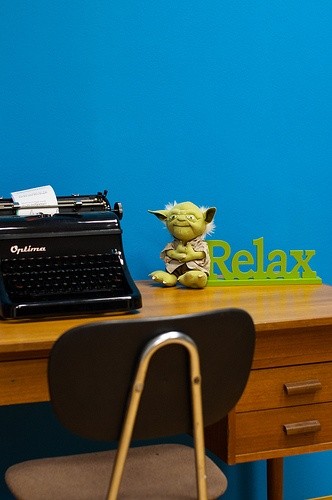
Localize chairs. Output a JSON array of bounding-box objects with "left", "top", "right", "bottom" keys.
[{"left": 4, "top": 306, "right": 257, "bottom": 499}]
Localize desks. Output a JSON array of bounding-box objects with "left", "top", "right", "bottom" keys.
[{"left": 0, "top": 272, "right": 331, "bottom": 500}]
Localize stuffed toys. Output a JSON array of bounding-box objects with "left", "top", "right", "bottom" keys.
[{"left": 147, "top": 200, "right": 218, "bottom": 289}]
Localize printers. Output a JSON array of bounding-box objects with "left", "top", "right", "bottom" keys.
[{"left": 1, "top": 185, "right": 143, "bottom": 317}]
